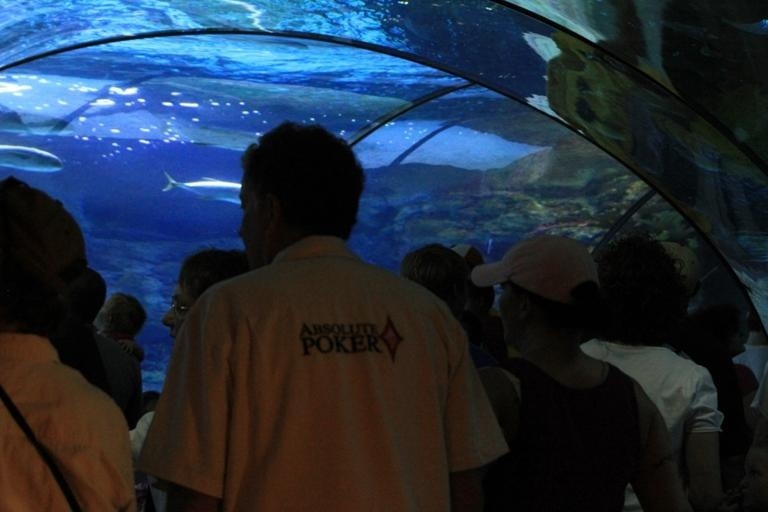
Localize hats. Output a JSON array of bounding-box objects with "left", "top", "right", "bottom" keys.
[
  {"left": 2, "top": 175, "right": 85, "bottom": 297},
  {"left": 472, "top": 233, "right": 601, "bottom": 305}
]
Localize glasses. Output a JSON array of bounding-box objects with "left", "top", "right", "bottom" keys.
[{"left": 170, "top": 298, "right": 189, "bottom": 319}]
